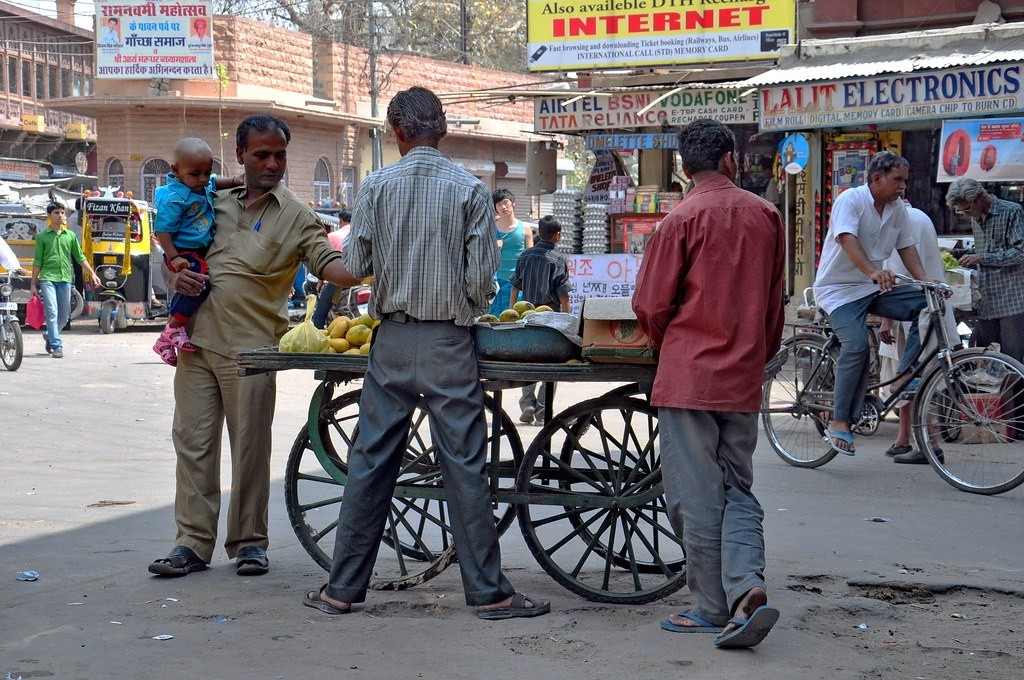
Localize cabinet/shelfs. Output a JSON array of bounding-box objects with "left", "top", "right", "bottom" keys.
[{"left": 734, "top": 127, "right": 780, "bottom": 193}]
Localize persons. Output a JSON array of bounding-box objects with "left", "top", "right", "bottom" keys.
[
  {"left": 300, "top": 86, "right": 555, "bottom": 620},
  {"left": 66, "top": 197, "right": 84, "bottom": 297},
  {"left": 311, "top": 212, "right": 352, "bottom": 329},
  {"left": 190, "top": 19, "right": 211, "bottom": 44},
  {"left": 946, "top": 177, "right": 1024, "bottom": 442},
  {"left": 30, "top": 202, "right": 101, "bottom": 357},
  {"left": 150, "top": 137, "right": 245, "bottom": 368},
  {"left": 0, "top": 235, "right": 29, "bottom": 277},
  {"left": 487, "top": 188, "right": 533, "bottom": 321},
  {"left": 814, "top": 153, "right": 954, "bottom": 456},
  {"left": 101, "top": 18, "right": 119, "bottom": 44},
  {"left": 881, "top": 195, "right": 960, "bottom": 463},
  {"left": 632, "top": 119, "right": 784, "bottom": 649},
  {"left": 146, "top": 114, "right": 363, "bottom": 577},
  {"left": 757, "top": 157, "right": 786, "bottom": 214},
  {"left": 506, "top": 214, "right": 572, "bottom": 427}
]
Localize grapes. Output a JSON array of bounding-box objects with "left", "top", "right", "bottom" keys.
[{"left": 939, "top": 250, "right": 958, "bottom": 270}]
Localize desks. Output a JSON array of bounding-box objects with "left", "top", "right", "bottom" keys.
[{"left": 610, "top": 211, "right": 668, "bottom": 254}]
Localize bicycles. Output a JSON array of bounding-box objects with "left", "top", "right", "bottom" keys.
[{"left": 759, "top": 272, "right": 1024, "bottom": 496}]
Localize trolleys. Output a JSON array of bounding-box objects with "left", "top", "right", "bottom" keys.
[{"left": 240, "top": 343, "right": 691, "bottom": 609}]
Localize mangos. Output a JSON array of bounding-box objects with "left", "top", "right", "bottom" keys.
[
  {"left": 319, "top": 314, "right": 382, "bottom": 355},
  {"left": 480, "top": 301, "right": 553, "bottom": 323},
  {"left": 567, "top": 359, "right": 582, "bottom": 365}
]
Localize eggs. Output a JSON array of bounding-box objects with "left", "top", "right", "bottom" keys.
[{"left": 554, "top": 189, "right": 610, "bottom": 254}]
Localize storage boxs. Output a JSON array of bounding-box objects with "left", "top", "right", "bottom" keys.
[
  {"left": 957, "top": 371, "right": 1019, "bottom": 444},
  {"left": 582, "top": 297, "right": 657, "bottom": 365}
]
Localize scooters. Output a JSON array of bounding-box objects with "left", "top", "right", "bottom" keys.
[{"left": 0, "top": 270, "right": 28, "bottom": 371}]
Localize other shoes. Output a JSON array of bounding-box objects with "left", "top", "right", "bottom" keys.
[
  {"left": 520, "top": 406, "right": 535, "bottom": 422},
  {"left": 535, "top": 421, "right": 544, "bottom": 426},
  {"left": 894, "top": 448, "right": 944, "bottom": 464},
  {"left": 53, "top": 347, "right": 63, "bottom": 358},
  {"left": 43, "top": 333, "right": 53, "bottom": 354},
  {"left": 886, "top": 443, "right": 913, "bottom": 457}
]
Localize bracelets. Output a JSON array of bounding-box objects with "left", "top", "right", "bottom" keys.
[
  {"left": 170, "top": 254, "right": 181, "bottom": 261},
  {"left": 232, "top": 176, "right": 236, "bottom": 185}
]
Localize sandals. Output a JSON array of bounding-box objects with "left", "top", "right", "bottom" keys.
[
  {"left": 153, "top": 338, "right": 177, "bottom": 366},
  {"left": 161, "top": 324, "right": 197, "bottom": 352}
]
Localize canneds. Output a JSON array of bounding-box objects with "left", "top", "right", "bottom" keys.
[{"left": 839, "top": 164, "right": 857, "bottom": 183}]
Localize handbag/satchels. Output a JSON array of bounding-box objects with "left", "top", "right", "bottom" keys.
[
  {"left": 27, "top": 295, "right": 44, "bottom": 330},
  {"left": 279, "top": 294, "right": 329, "bottom": 353}
]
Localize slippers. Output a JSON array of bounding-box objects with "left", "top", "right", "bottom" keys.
[
  {"left": 824, "top": 429, "right": 855, "bottom": 456},
  {"left": 715, "top": 606, "right": 780, "bottom": 647},
  {"left": 148, "top": 546, "right": 207, "bottom": 576},
  {"left": 893, "top": 379, "right": 941, "bottom": 398},
  {"left": 478, "top": 591, "right": 550, "bottom": 618},
  {"left": 236, "top": 546, "right": 269, "bottom": 574},
  {"left": 661, "top": 609, "right": 725, "bottom": 632},
  {"left": 303, "top": 584, "right": 343, "bottom": 614}
]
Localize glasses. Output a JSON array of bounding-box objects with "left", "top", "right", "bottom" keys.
[{"left": 956, "top": 201, "right": 973, "bottom": 214}]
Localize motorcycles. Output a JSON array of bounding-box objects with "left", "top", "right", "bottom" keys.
[{"left": 74, "top": 190, "right": 168, "bottom": 334}]
